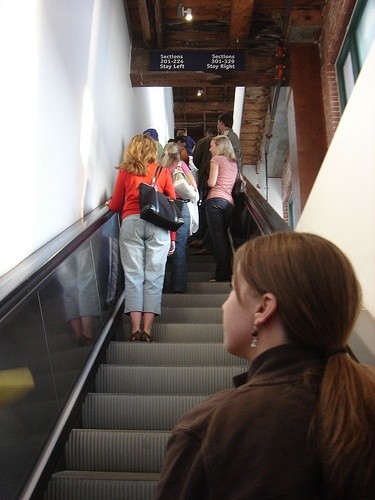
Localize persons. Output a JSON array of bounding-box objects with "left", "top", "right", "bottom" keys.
[
  {"left": 190, "top": 127, "right": 218, "bottom": 254},
  {"left": 106, "top": 133, "right": 175, "bottom": 342},
  {"left": 217, "top": 114, "right": 240, "bottom": 160},
  {"left": 205, "top": 138, "right": 238, "bottom": 282},
  {"left": 155, "top": 232, "right": 375, "bottom": 500},
  {"left": 58, "top": 229, "right": 103, "bottom": 347},
  {"left": 163, "top": 143, "right": 199, "bottom": 293},
  {"left": 168, "top": 129, "right": 198, "bottom": 177},
  {"left": 143, "top": 129, "right": 164, "bottom": 162}
]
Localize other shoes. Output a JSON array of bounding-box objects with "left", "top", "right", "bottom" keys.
[
  {"left": 163, "top": 290, "right": 184, "bottom": 294},
  {"left": 208, "top": 278, "right": 218, "bottom": 283},
  {"left": 128, "top": 330, "right": 153, "bottom": 343},
  {"left": 76, "top": 335, "right": 96, "bottom": 348},
  {"left": 191, "top": 248, "right": 212, "bottom": 255},
  {"left": 189, "top": 240, "right": 205, "bottom": 247}
]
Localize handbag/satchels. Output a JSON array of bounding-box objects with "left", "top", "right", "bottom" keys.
[
  {"left": 139, "top": 166, "right": 185, "bottom": 232},
  {"left": 172, "top": 160, "right": 200, "bottom": 204},
  {"left": 231, "top": 166, "right": 244, "bottom": 196}
]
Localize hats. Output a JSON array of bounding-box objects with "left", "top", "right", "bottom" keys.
[{"left": 143, "top": 129, "right": 158, "bottom": 141}]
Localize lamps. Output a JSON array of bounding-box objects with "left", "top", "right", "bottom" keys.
[
  {"left": 182, "top": 7, "right": 192, "bottom": 21},
  {"left": 197, "top": 90, "right": 203, "bottom": 96}
]
